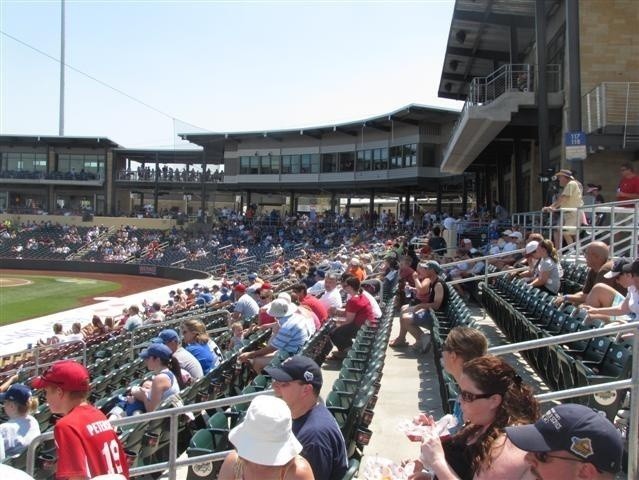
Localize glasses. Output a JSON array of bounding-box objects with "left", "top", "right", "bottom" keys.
[
  {"left": 458, "top": 390, "right": 496, "bottom": 402},
  {"left": 441, "top": 344, "right": 458, "bottom": 354}
]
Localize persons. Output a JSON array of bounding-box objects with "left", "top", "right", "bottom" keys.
[
  {"left": 516, "top": 68, "right": 534, "bottom": 91},
  {"left": 155, "top": 329, "right": 204, "bottom": 388},
  {"left": 345, "top": 259, "right": 365, "bottom": 282},
  {"left": 396, "top": 255, "right": 416, "bottom": 289},
  {"left": 44, "top": 361, "right": 129, "bottom": 476},
  {"left": 94, "top": 202, "right": 448, "bottom": 228},
  {"left": 176, "top": 318, "right": 214, "bottom": 376},
  {"left": 582, "top": 258, "right": 634, "bottom": 322},
  {"left": 273, "top": 354, "right": 350, "bottom": 476},
  {"left": 0, "top": 383, "right": 42, "bottom": 470},
  {"left": 316, "top": 226, "right": 431, "bottom": 264},
  {"left": 107, "top": 343, "right": 180, "bottom": 422},
  {"left": 553, "top": 241, "right": 611, "bottom": 310},
  {"left": 0, "top": 374, "right": 19, "bottom": 393},
  {"left": 123, "top": 306, "right": 143, "bottom": 332},
  {"left": 259, "top": 277, "right": 296, "bottom": 299},
  {"left": 542, "top": 170, "right": 584, "bottom": 256},
  {"left": 616, "top": 163, "right": 639, "bottom": 259},
  {"left": 444, "top": 214, "right": 462, "bottom": 229},
  {"left": 118, "top": 162, "right": 223, "bottom": 184},
  {"left": 420, "top": 356, "right": 535, "bottom": 476},
  {"left": 429, "top": 227, "right": 447, "bottom": 260},
  {"left": 141, "top": 299, "right": 166, "bottom": 324},
  {"left": 177, "top": 225, "right": 320, "bottom": 260},
  {"left": 527, "top": 239, "right": 560, "bottom": 296},
  {"left": 102, "top": 223, "right": 182, "bottom": 266},
  {"left": 238, "top": 298, "right": 307, "bottom": 378},
  {"left": 157, "top": 278, "right": 250, "bottom": 316},
  {"left": 62, "top": 323, "right": 84, "bottom": 341},
  {"left": 246, "top": 274, "right": 262, "bottom": 296},
  {"left": 306, "top": 269, "right": 326, "bottom": 296},
  {"left": 365, "top": 256, "right": 396, "bottom": 289},
  {"left": 448, "top": 228, "right": 542, "bottom": 275},
  {"left": 214, "top": 258, "right": 315, "bottom": 280},
  {"left": 413, "top": 325, "right": 488, "bottom": 438},
  {"left": 218, "top": 395, "right": 313, "bottom": 476},
  {"left": 328, "top": 276, "right": 375, "bottom": 357},
  {"left": 459, "top": 198, "right": 500, "bottom": 224},
  {"left": 324, "top": 158, "right": 356, "bottom": 173},
  {"left": 487, "top": 215, "right": 501, "bottom": 229},
  {"left": 1, "top": 166, "right": 99, "bottom": 182},
  {"left": 491, "top": 200, "right": 509, "bottom": 222},
  {"left": 525, "top": 240, "right": 541, "bottom": 259},
  {"left": 37, "top": 323, "right": 65, "bottom": 347},
  {"left": 227, "top": 321, "right": 255, "bottom": 352},
  {"left": 116, "top": 308, "right": 129, "bottom": 327},
  {"left": 390, "top": 261, "right": 449, "bottom": 354},
  {"left": 318, "top": 275, "right": 342, "bottom": 310},
  {"left": 576, "top": 257, "right": 638, "bottom": 343},
  {"left": 84, "top": 316, "right": 109, "bottom": 340},
  {"left": 291, "top": 284, "right": 330, "bottom": 325},
  {"left": 0, "top": 218, "right": 109, "bottom": 261},
  {"left": 1, "top": 193, "right": 94, "bottom": 217},
  {"left": 580, "top": 184, "right": 606, "bottom": 242},
  {"left": 451, "top": 251, "right": 485, "bottom": 306},
  {"left": 524, "top": 403, "right": 625, "bottom": 475},
  {"left": 197, "top": 322, "right": 223, "bottom": 367},
  {"left": 400, "top": 261, "right": 432, "bottom": 314},
  {"left": 226, "top": 284, "right": 260, "bottom": 330}
]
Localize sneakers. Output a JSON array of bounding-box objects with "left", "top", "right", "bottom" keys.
[{"left": 418, "top": 334, "right": 431, "bottom": 355}]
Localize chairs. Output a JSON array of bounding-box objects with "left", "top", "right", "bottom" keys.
[
  {"left": 472, "top": 262, "right": 635, "bottom": 428},
  {"left": 430, "top": 283, "right": 492, "bottom": 419},
  {"left": 0, "top": 307, "right": 335, "bottom": 480},
  {"left": 326, "top": 293, "right": 400, "bottom": 478}
]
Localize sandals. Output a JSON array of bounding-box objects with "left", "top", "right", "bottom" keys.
[{"left": 389, "top": 339, "right": 409, "bottom": 348}]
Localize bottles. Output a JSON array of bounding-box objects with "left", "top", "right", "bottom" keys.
[
  {"left": 405, "top": 281, "right": 412, "bottom": 297},
  {"left": 237, "top": 357, "right": 244, "bottom": 372}
]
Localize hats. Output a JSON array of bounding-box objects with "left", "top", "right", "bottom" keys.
[
  {"left": 153, "top": 329, "right": 179, "bottom": 343},
  {"left": 554, "top": 168, "right": 574, "bottom": 180},
  {"left": 461, "top": 238, "right": 472, "bottom": 245},
  {"left": 139, "top": 341, "right": 172, "bottom": 363},
  {"left": 418, "top": 259, "right": 442, "bottom": 276},
  {"left": 247, "top": 273, "right": 257, "bottom": 280},
  {"left": 603, "top": 258, "right": 631, "bottom": 279},
  {"left": 509, "top": 232, "right": 523, "bottom": 240},
  {"left": 233, "top": 284, "right": 247, "bottom": 292},
  {"left": 277, "top": 292, "right": 291, "bottom": 304},
  {"left": 488, "top": 231, "right": 499, "bottom": 240},
  {"left": 444, "top": 217, "right": 457, "bottom": 229},
  {"left": 623, "top": 258, "right": 639, "bottom": 275},
  {"left": 227, "top": 393, "right": 304, "bottom": 467},
  {"left": 265, "top": 298, "right": 293, "bottom": 318},
  {"left": 31, "top": 358, "right": 90, "bottom": 393},
  {"left": 262, "top": 354, "right": 323, "bottom": 386},
  {"left": 526, "top": 241, "right": 539, "bottom": 254},
  {"left": 502, "top": 401, "right": 623, "bottom": 475},
  {"left": 0, "top": 382, "right": 33, "bottom": 406},
  {"left": 503, "top": 229, "right": 513, "bottom": 235}
]
